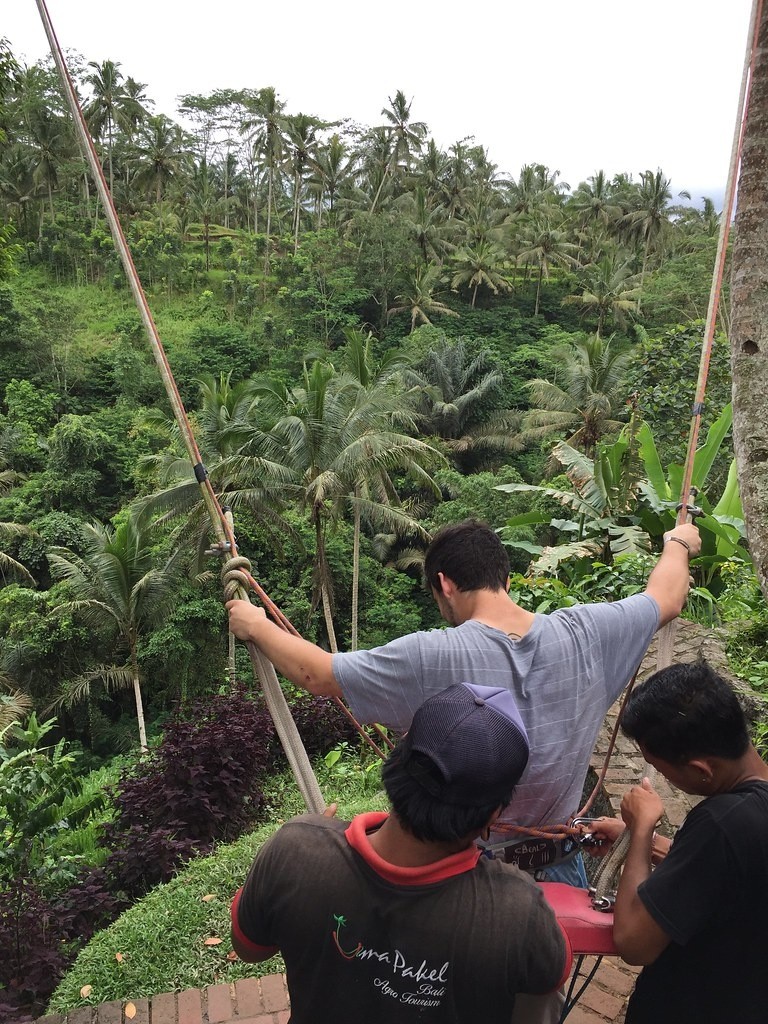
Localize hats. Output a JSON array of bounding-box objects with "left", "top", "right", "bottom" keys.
[{"left": 403, "top": 683, "right": 530, "bottom": 797}]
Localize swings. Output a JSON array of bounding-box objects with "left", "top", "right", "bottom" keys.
[{"left": 27, "top": 0, "right": 755, "bottom": 1024}]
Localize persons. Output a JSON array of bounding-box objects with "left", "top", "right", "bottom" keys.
[
  {"left": 223, "top": 519, "right": 703, "bottom": 889},
  {"left": 580, "top": 662, "right": 768, "bottom": 1024},
  {"left": 228, "top": 683, "right": 574, "bottom": 1024}
]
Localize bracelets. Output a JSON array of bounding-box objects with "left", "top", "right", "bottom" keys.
[
  {"left": 670, "top": 537, "right": 690, "bottom": 553},
  {"left": 650, "top": 831, "right": 658, "bottom": 858}
]
